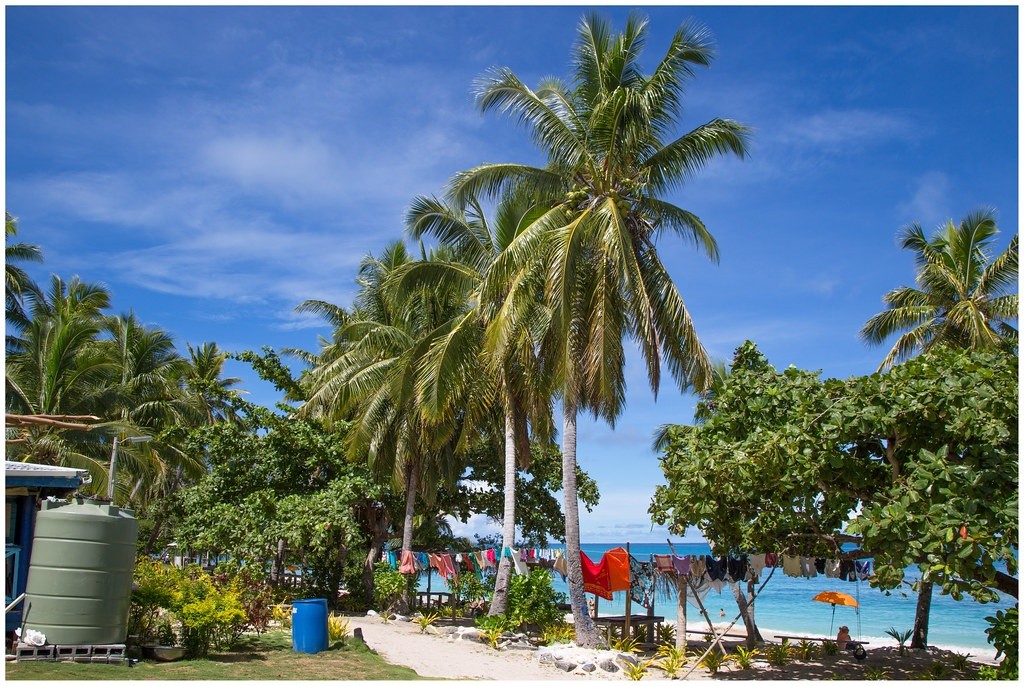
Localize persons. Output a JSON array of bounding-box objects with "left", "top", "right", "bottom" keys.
[
  {"left": 589, "top": 597, "right": 595, "bottom": 616},
  {"left": 720, "top": 608, "right": 725, "bottom": 616},
  {"left": 837, "top": 625, "right": 851, "bottom": 648}
]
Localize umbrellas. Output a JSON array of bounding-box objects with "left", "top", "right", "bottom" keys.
[{"left": 812, "top": 591, "right": 860, "bottom": 636}]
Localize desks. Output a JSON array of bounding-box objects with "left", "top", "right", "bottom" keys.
[
  {"left": 417, "top": 592, "right": 451, "bottom": 609},
  {"left": 592, "top": 615, "right": 665, "bottom": 643}
]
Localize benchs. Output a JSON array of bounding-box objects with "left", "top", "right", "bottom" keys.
[{"left": 774, "top": 635, "right": 869, "bottom": 654}]
